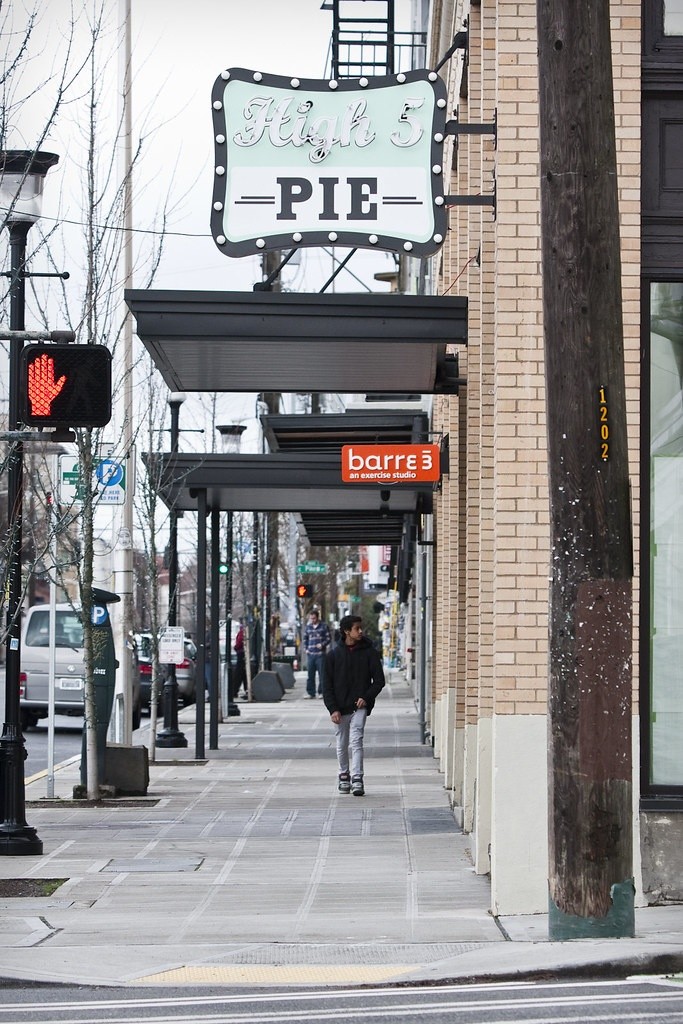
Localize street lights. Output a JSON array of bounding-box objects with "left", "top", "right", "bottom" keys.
[
  {"left": 1, "top": 137, "right": 56, "bottom": 860},
  {"left": 56, "top": 449, "right": 125, "bottom": 506}
]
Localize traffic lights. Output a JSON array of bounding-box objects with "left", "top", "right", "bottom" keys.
[
  {"left": 297, "top": 583, "right": 312, "bottom": 598},
  {"left": 16, "top": 336, "right": 115, "bottom": 429}
]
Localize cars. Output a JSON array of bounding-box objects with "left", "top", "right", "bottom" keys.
[{"left": 132, "top": 628, "right": 203, "bottom": 716}]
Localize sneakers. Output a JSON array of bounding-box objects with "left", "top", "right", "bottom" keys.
[
  {"left": 351, "top": 775, "right": 365, "bottom": 796},
  {"left": 338, "top": 772, "right": 351, "bottom": 794}
]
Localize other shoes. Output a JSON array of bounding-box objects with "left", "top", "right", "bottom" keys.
[{"left": 303, "top": 694, "right": 323, "bottom": 699}]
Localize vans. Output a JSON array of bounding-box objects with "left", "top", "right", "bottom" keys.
[{"left": 13, "top": 595, "right": 143, "bottom": 730}]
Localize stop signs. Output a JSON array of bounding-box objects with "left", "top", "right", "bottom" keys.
[{"left": 90, "top": 603, "right": 109, "bottom": 626}]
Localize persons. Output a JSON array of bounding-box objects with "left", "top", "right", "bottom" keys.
[
  {"left": 201, "top": 626, "right": 216, "bottom": 702},
  {"left": 330, "top": 623, "right": 339, "bottom": 644},
  {"left": 233, "top": 624, "right": 251, "bottom": 700},
  {"left": 373, "top": 633, "right": 383, "bottom": 662},
  {"left": 274, "top": 620, "right": 281, "bottom": 650},
  {"left": 305, "top": 611, "right": 330, "bottom": 702},
  {"left": 285, "top": 628, "right": 294, "bottom": 647},
  {"left": 321, "top": 615, "right": 385, "bottom": 795}
]
